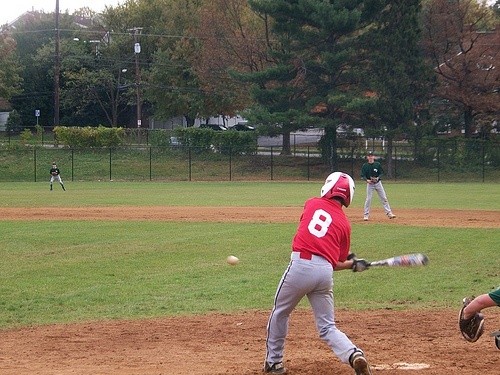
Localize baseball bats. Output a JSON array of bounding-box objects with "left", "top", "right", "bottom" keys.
[{"left": 365, "top": 253, "right": 429, "bottom": 267}]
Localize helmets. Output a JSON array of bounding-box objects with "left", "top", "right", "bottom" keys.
[{"left": 319, "top": 170, "right": 356, "bottom": 209}]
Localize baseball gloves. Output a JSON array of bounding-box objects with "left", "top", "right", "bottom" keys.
[
  {"left": 52, "top": 172, "right": 58, "bottom": 177},
  {"left": 369, "top": 176, "right": 380, "bottom": 184},
  {"left": 457, "top": 296, "right": 486, "bottom": 343}
]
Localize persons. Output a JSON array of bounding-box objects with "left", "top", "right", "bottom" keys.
[
  {"left": 49, "top": 162, "right": 66, "bottom": 191},
  {"left": 263, "top": 172, "right": 372, "bottom": 375},
  {"left": 458, "top": 286, "right": 500, "bottom": 349},
  {"left": 360, "top": 151, "right": 393, "bottom": 217}
]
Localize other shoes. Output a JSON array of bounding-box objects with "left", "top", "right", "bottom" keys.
[
  {"left": 363, "top": 215, "right": 369, "bottom": 221},
  {"left": 388, "top": 212, "right": 396, "bottom": 219}
]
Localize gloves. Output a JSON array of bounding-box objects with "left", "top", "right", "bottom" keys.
[
  {"left": 346, "top": 253, "right": 357, "bottom": 261},
  {"left": 351, "top": 258, "right": 370, "bottom": 273}
]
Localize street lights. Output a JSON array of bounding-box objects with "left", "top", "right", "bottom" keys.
[{"left": 126, "top": 26, "right": 144, "bottom": 144}]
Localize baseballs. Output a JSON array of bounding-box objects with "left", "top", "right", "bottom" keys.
[{"left": 226, "top": 255, "right": 239, "bottom": 266}]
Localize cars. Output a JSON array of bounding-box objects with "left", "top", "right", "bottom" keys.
[
  {"left": 198, "top": 124, "right": 229, "bottom": 131},
  {"left": 229, "top": 122, "right": 255, "bottom": 131},
  {"left": 167, "top": 136, "right": 182, "bottom": 147}
]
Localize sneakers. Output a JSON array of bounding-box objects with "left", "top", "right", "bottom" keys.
[
  {"left": 349, "top": 348, "right": 372, "bottom": 375},
  {"left": 263, "top": 358, "right": 286, "bottom": 374}
]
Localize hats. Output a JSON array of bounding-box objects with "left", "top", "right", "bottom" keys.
[{"left": 367, "top": 150, "right": 375, "bottom": 156}]
